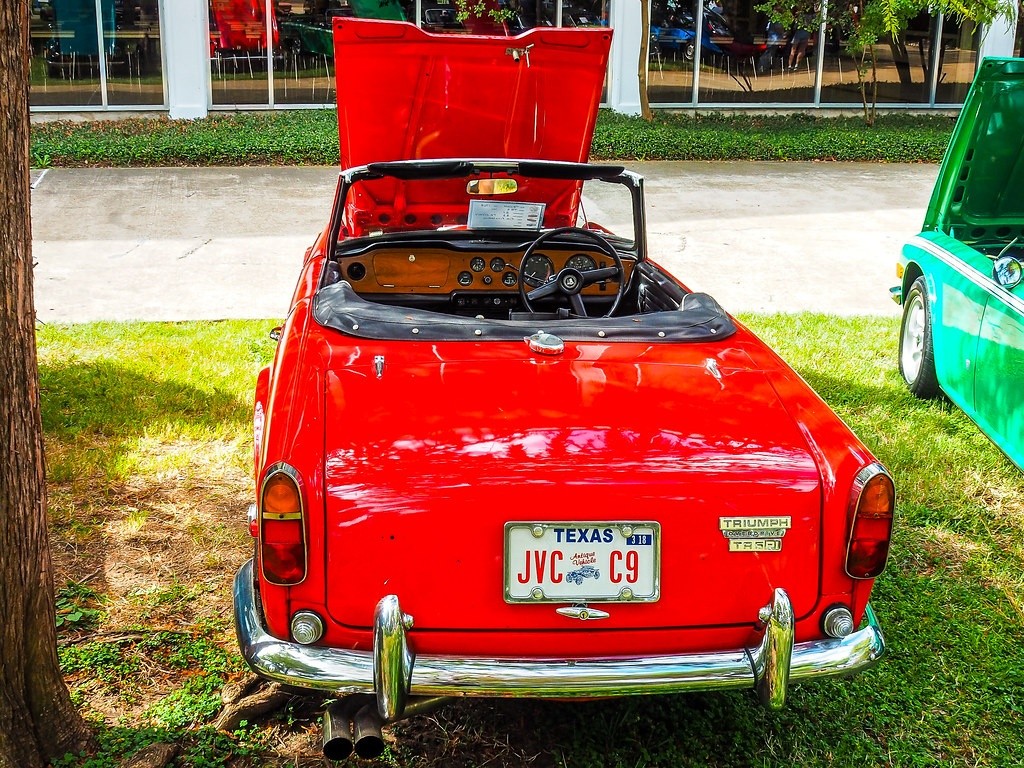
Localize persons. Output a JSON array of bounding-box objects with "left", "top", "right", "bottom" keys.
[{"left": 726, "top": 6, "right": 817, "bottom": 72}]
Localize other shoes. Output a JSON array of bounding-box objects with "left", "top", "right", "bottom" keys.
[
  {"left": 788, "top": 68, "right": 793, "bottom": 72},
  {"left": 794, "top": 67, "right": 798, "bottom": 72}
]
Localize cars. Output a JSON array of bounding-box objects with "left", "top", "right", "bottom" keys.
[
  {"left": 233, "top": 16, "right": 895, "bottom": 758},
  {"left": 899, "top": 54, "right": 1024, "bottom": 485},
  {"left": 650, "top": 4, "right": 731, "bottom": 61}
]
